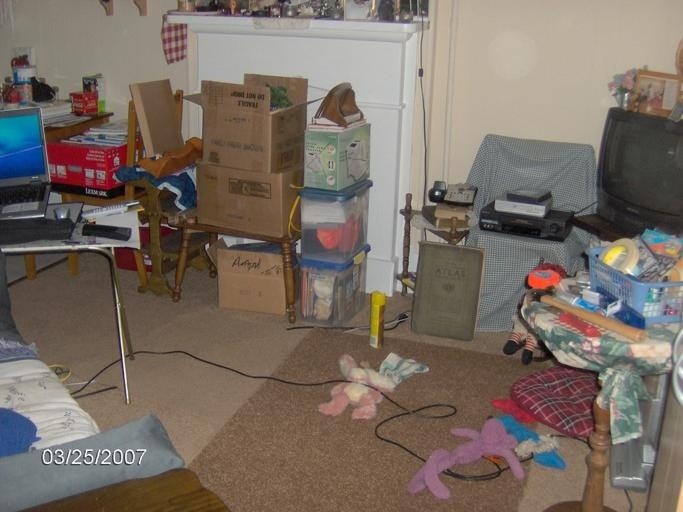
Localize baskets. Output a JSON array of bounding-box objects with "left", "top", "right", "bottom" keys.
[{"left": 584, "top": 247, "right": 683, "bottom": 326}]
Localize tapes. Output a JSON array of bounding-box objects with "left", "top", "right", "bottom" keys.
[{"left": 596, "top": 237, "right": 640, "bottom": 281}]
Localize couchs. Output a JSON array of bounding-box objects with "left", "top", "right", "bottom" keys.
[{"left": 0, "top": 250, "right": 183, "bottom": 511}]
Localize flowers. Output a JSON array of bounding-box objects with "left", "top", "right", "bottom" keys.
[{"left": 606, "top": 66, "right": 638, "bottom": 96}]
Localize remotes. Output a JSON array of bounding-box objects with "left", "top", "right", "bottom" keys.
[
  {"left": 81, "top": 202, "right": 127, "bottom": 219},
  {"left": 82, "top": 224, "right": 132, "bottom": 241}
]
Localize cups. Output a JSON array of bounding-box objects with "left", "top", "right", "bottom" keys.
[{"left": 574, "top": 270, "right": 591, "bottom": 291}]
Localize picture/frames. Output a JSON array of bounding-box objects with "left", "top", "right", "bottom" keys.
[{"left": 630, "top": 69, "right": 683, "bottom": 119}]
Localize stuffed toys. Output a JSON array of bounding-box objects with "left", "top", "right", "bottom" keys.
[
  {"left": 316, "top": 351, "right": 388, "bottom": 421},
  {"left": 504, "top": 311, "right": 539, "bottom": 364},
  {"left": 407, "top": 410, "right": 523, "bottom": 498}
]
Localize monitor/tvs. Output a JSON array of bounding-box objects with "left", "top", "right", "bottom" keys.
[{"left": 596, "top": 106, "right": 683, "bottom": 235}]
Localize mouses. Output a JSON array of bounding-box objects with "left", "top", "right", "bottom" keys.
[{"left": 53, "top": 206, "right": 70, "bottom": 220}]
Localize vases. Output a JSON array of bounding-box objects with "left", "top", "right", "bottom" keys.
[{"left": 617, "top": 92, "right": 629, "bottom": 108}]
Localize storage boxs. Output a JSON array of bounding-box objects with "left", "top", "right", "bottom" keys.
[
  {"left": 179, "top": 72, "right": 325, "bottom": 174},
  {"left": 45, "top": 134, "right": 144, "bottom": 201},
  {"left": 202, "top": 238, "right": 301, "bottom": 317},
  {"left": 296, "top": 244, "right": 371, "bottom": 326},
  {"left": 193, "top": 159, "right": 304, "bottom": 240},
  {"left": 302, "top": 121, "right": 371, "bottom": 192},
  {"left": 295, "top": 180, "right": 373, "bottom": 263}
]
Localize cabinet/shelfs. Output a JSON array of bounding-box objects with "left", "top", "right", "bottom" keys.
[{"left": 395, "top": 193, "right": 469, "bottom": 296}]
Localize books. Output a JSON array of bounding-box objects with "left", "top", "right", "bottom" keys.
[{"left": 20, "top": 99, "right": 140, "bottom": 148}]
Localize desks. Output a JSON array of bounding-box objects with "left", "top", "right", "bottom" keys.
[
  {"left": 522, "top": 294, "right": 682, "bottom": 511},
  {"left": 573, "top": 213, "right": 636, "bottom": 244},
  {"left": 166, "top": 205, "right": 304, "bottom": 324},
  {"left": 23, "top": 110, "right": 114, "bottom": 279}
]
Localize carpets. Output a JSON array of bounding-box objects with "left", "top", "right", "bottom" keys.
[{"left": 188, "top": 328, "right": 546, "bottom": 511}]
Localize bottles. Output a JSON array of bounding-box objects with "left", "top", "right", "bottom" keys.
[{"left": 368, "top": 290, "right": 385, "bottom": 350}]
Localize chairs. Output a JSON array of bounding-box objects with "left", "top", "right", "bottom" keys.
[{"left": 58, "top": 88, "right": 185, "bottom": 295}]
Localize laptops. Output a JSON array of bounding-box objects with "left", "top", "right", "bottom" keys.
[{"left": 0, "top": 108, "right": 52, "bottom": 221}]
